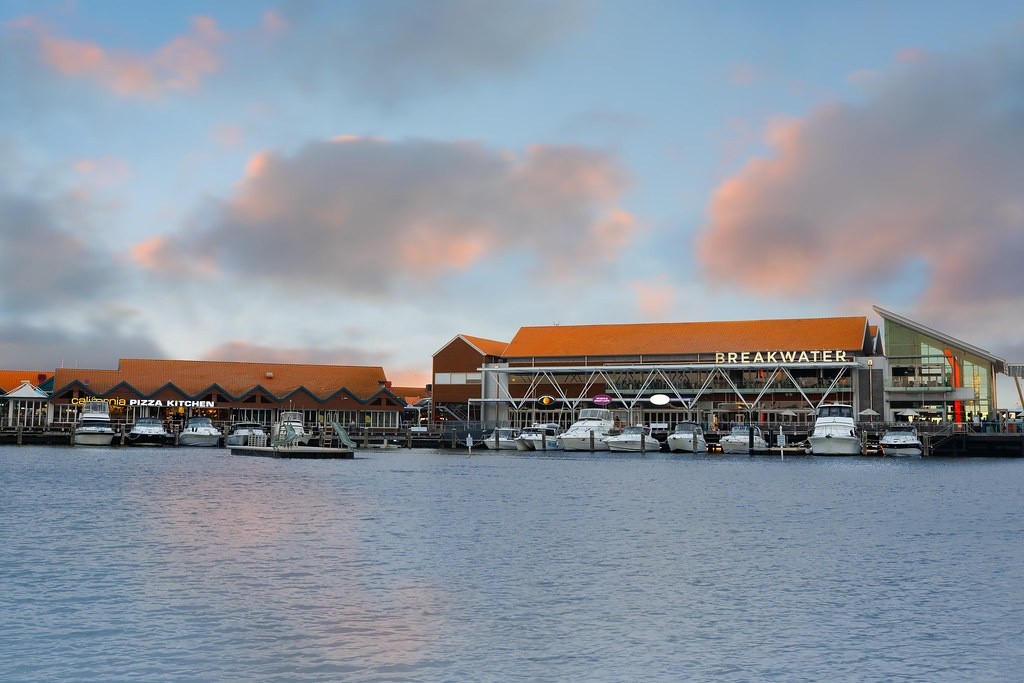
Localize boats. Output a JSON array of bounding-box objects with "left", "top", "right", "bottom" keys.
[
  {"left": 69, "top": 402, "right": 116, "bottom": 446},
  {"left": 483, "top": 422, "right": 565, "bottom": 452},
  {"left": 177, "top": 417, "right": 223, "bottom": 448},
  {"left": 224, "top": 398, "right": 311, "bottom": 448},
  {"left": 122, "top": 418, "right": 168, "bottom": 448},
  {"left": 719, "top": 423, "right": 768, "bottom": 454},
  {"left": 560, "top": 408, "right": 624, "bottom": 451},
  {"left": 603, "top": 423, "right": 662, "bottom": 452},
  {"left": 667, "top": 420, "right": 707, "bottom": 452},
  {"left": 807, "top": 400, "right": 862, "bottom": 456},
  {"left": 879, "top": 426, "right": 923, "bottom": 457}
]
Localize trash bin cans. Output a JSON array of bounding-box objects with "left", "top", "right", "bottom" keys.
[{"left": 1008, "top": 419, "right": 1016, "bottom": 432}]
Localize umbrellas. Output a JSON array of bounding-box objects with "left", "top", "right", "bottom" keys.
[
  {"left": 858, "top": 408, "right": 880, "bottom": 415},
  {"left": 897, "top": 409, "right": 920, "bottom": 416},
  {"left": 778, "top": 409, "right": 797, "bottom": 416}
]
